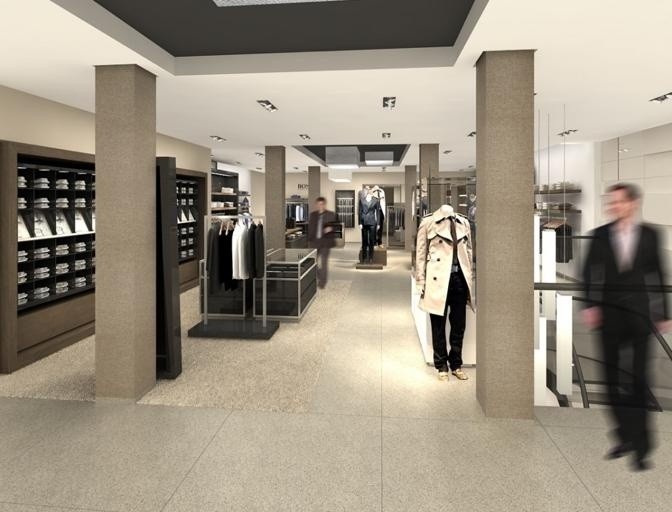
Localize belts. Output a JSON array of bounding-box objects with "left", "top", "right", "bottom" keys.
[{"left": 452, "top": 265, "right": 460, "bottom": 273}]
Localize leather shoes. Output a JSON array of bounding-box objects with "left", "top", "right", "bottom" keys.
[
  {"left": 439, "top": 368, "right": 468, "bottom": 381},
  {"left": 605, "top": 442, "right": 648, "bottom": 470}
]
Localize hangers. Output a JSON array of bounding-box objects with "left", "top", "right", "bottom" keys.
[{"left": 541, "top": 217, "right": 568, "bottom": 229}]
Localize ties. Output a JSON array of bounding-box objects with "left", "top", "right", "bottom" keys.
[
  {"left": 317, "top": 215, "right": 322, "bottom": 241},
  {"left": 448, "top": 216, "right": 459, "bottom": 264}
]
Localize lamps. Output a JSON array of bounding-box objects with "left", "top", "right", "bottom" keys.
[
  {"left": 325, "top": 145, "right": 361, "bottom": 170},
  {"left": 364, "top": 151, "right": 394, "bottom": 166}
]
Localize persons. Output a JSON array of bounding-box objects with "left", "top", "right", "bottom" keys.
[
  {"left": 307, "top": 197, "right": 337, "bottom": 290},
  {"left": 577, "top": 182, "right": 671, "bottom": 471},
  {"left": 359, "top": 184, "right": 386, "bottom": 264},
  {"left": 415, "top": 203, "right": 476, "bottom": 383}
]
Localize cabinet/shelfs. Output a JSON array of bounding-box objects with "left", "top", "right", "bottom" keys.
[{"left": 534, "top": 189, "right": 582, "bottom": 214}]
